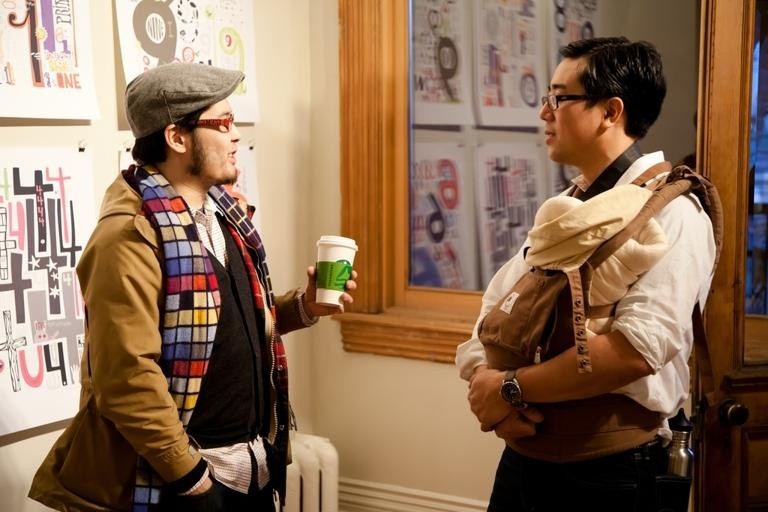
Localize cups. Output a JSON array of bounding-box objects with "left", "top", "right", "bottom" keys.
[{"left": 314, "top": 233, "right": 358, "bottom": 309}]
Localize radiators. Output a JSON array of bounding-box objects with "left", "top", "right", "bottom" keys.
[{"left": 283, "top": 427, "right": 342, "bottom": 510}]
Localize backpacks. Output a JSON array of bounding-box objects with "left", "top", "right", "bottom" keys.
[{"left": 476, "top": 163, "right": 726, "bottom": 459}]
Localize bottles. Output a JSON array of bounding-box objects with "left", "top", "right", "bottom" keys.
[{"left": 665, "top": 406, "right": 694, "bottom": 482}]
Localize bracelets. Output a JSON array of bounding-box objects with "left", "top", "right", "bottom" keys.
[{"left": 500, "top": 367, "right": 530, "bottom": 411}]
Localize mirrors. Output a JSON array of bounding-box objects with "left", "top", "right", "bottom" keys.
[{"left": 339, "top": 0, "right": 706, "bottom": 367}]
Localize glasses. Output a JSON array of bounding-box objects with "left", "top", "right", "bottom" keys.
[
  {"left": 541, "top": 94, "right": 588, "bottom": 112},
  {"left": 186, "top": 114, "right": 234, "bottom": 133}
]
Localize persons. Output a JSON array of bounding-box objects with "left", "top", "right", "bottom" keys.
[
  {"left": 23, "top": 63, "right": 357, "bottom": 512},
  {"left": 455, "top": 37, "right": 725, "bottom": 511}
]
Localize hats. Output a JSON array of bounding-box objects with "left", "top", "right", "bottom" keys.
[{"left": 123, "top": 62, "right": 247, "bottom": 140}]
